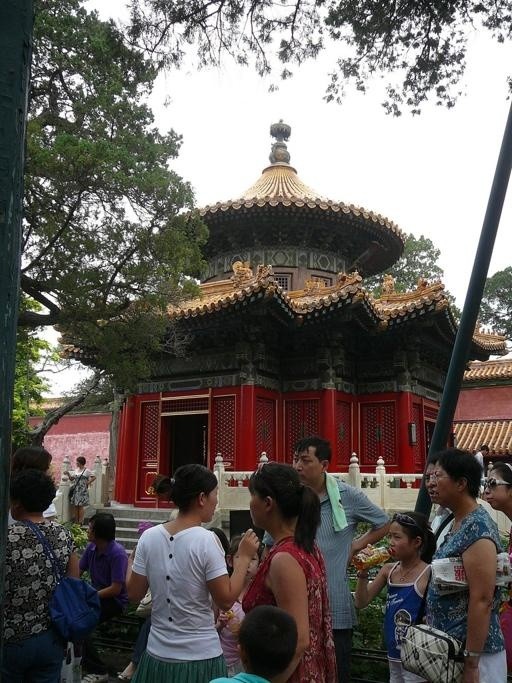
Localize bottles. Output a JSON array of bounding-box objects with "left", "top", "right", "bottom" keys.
[
  {"left": 229, "top": 610, "right": 242, "bottom": 643},
  {"left": 352, "top": 546, "right": 394, "bottom": 570}
]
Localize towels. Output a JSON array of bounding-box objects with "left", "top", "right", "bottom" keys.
[{"left": 325, "top": 471, "right": 348, "bottom": 533}]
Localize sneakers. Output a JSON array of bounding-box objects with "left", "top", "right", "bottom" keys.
[
  {"left": 119, "top": 669, "right": 132, "bottom": 680},
  {"left": 81, "top": 675, "right": 108, "bottom": 683}
]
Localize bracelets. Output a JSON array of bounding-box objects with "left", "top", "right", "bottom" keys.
[{"left": 462, "top": 647, "right": 482, "bottom": 659}]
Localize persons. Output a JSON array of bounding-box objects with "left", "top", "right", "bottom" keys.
[
  {"left": 485, "top": 459, "right": 512, "bottom": 682},
  {"left": 474, "top": 445, "right": 490, "bottom": 472},
  {"left": 353, "top": 510, "right": 436, "bottom": 682},
  {"left": 427, "top": 448, "right": 508, "bottom": 683},
  {"left": 117, "top": 434, "right": 393, "bottom": 681},
  {"left": 424, "top": 452, "right": 454, "bottom": 548},
  {"left": 2, "top": 447, "right": 128, "bottom": 681}
]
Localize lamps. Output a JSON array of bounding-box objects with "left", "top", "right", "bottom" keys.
[
  {"left": 451, "top": 432, "right": 457, "bottom": 448},
  {"left": 411, "top": 421, "right": 417, "bottom": 446}
]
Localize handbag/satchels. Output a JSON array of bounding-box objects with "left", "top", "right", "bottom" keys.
[
  {"left": 62, "top": 642, "right": 83, "bottom": 683},
  {"left": 50, "top": 578, "right": 100, "bottom": 640},
  {"left": 137, "top": 594, "right": 152, "bottom": 616},
  {"left": 68, "top": 488, "right": 74, "bottom": 499},
  {"left": 400, "top": 624, "right": 465, "bottom": 682}
]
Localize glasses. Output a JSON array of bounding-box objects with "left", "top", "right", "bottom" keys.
[
  {"left": 433, "top": 475, "right": 450, "bottom": 481},
  {"left": 393, "top": 513, "right": 419, "bottom": 527},
  {"left": 484, "top": 477, "right": 509, "bottom": 487}
]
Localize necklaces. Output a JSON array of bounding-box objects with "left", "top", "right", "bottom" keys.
[{"left": 397, "top": 565, "right": 419, "bottom": 583}]
